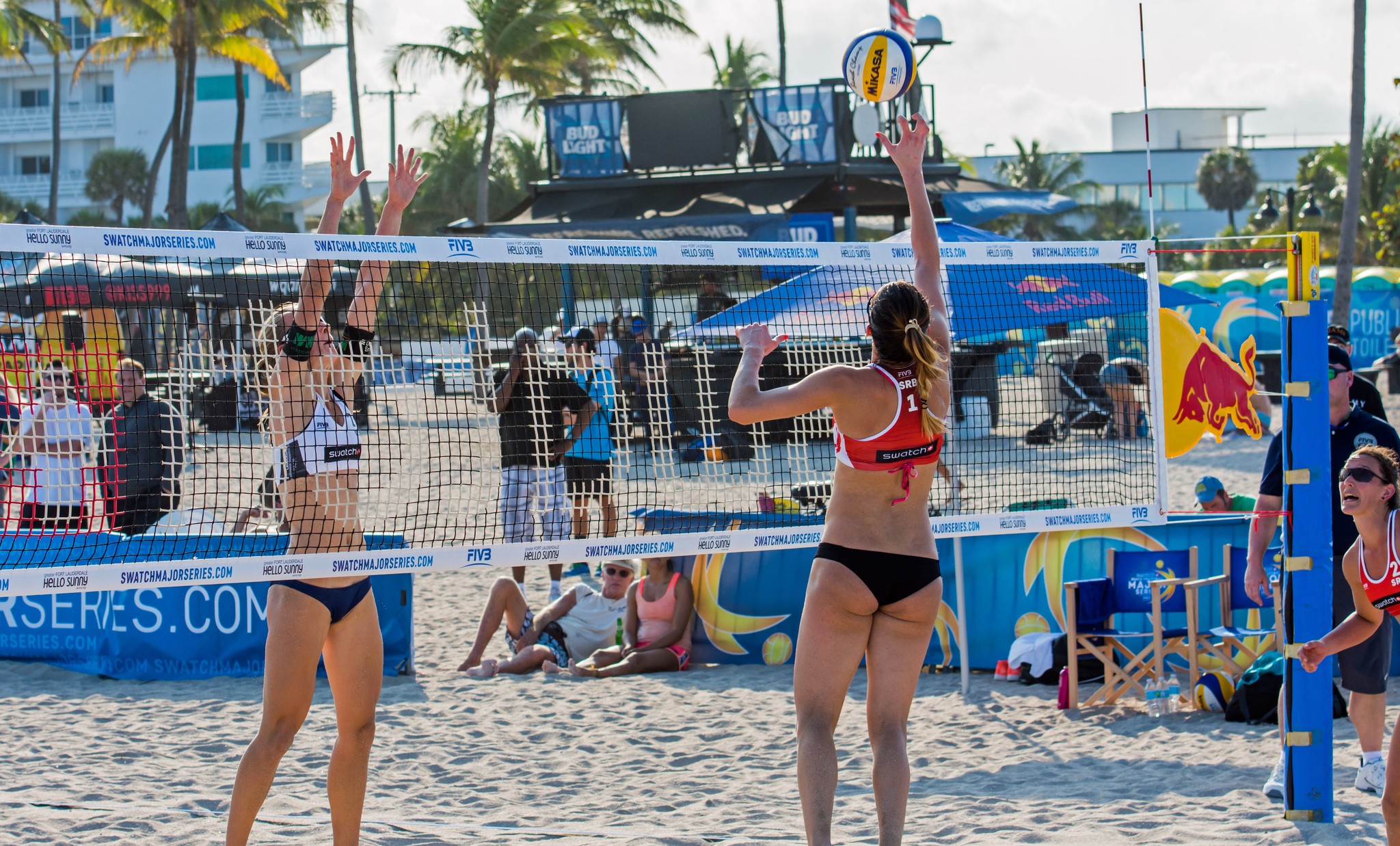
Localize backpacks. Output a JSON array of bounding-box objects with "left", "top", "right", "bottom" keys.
[{"left": 1226, "top": 651, "right": 1348, "bottom": 725}]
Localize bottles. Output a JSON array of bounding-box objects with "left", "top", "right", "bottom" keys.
[
  {"left": 1056, "top": 666, "right": 1070, "bottom": 710},
  {"left": 1167, "top": 674, "right": 1181, "bottom": 712},
  {"left": 614, "top": 617, "right": 623, "bottom": 645},
  {"left": 1144, "top": 678, "right": 1161, "bottom": 717},
  {"left": 1157, "top": 676, "right": 1172, "bottom": 716}
]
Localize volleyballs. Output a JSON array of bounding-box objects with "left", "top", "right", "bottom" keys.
[
  {"left": 1193, "top": 671, "right": 1236, "bottom": 715},
  {"left": 840, "top": 28, "right": 915, "bottom": 104}
]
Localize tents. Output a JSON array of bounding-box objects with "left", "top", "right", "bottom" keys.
[{"left": 0, "top": 207, "right": 359, "bottom": 433}]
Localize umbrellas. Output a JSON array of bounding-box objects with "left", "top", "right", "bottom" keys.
[{"left": 677, "top": 215, "right": 1218, "bottom": 697}]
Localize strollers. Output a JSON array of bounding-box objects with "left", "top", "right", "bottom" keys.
[{"left": 1044, "top": 348, "right": 1123, "bottom": 442}]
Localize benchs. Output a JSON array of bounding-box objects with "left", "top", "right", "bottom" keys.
[
  {"left": 148, "top": 391, "right": 185, "bottom": 407},
  {"left": 421, "top": 372, "right": 497, "bottom": 378}
]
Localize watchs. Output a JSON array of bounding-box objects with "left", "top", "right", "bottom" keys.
[{"left": 565, "top": 432, "right": 577, "bottom": 445}]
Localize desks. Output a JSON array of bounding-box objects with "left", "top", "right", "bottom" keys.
[
  {"left": 145, "top": 372, "right": 211, "bottom": 452},
  {"left": 425, "top": 359, "right": 473, "bottom": 397}
]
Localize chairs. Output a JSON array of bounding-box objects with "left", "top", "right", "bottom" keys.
[
  {"left": 1063, "top": 546, "right": 1199, "bottom": 706},
  {"left": 1183, "top": 543, "right": 1284, "bottom": 711}
]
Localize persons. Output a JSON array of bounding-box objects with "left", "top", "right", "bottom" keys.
[
  {"left": 229, "top": 466, "right": 291, "bottom": 533},
  {"left": 459, "top": 529, "right": 695, "bottom": 680},
  {"left": 0, "top": 359, "right": 184, "bottom": 536},
  {"left": 1097, "top": 352, "right": 1149, "bottom": 440},
  {"left": 725, "top": 112, "right": 953, "bottom": 846},
  {"left": 1191, "top": 472, "right": 1255, "bottom": 513},
  {"left": 696, "top": 270, "right": 737, "bottom": 323},
  {"left": 226, "top": 130, "right": 429, "bottom": 846},
  {"left": 484, "top": 305, "right": 673, "bottom": 607},
  {"left": 1243, "top": 324, "right": 1400, "bottom": 846}
]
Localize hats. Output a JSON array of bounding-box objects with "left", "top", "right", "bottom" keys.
[
  {"left": 593, "top": 315, "right": 609, "bottom": 325},
  {"left": 621, "top": 304, "right": 631, "bottom": 318},
  {"left": 1328, "top": 342, "right": 1353, "bottom": 371},
  {"left": 1327, "top": 325, "right": 1351, "bottom": 345},
  {"left": 631, "top": 312, "right": 640, "bottom": 317},
  {"left": 629, "top": 319, "right": 646, "bottom": 333},
  {"left": 558, "top": 325, "right": 595, "bottom": 346},
  {"left": 618, "top": 319, "right": 632, "bottom": 331},
  {"left": 1194, "top": 476, "right": 1224, "bottom": 506},
  {"left": 600, "top": 559, "right": 635, "bottom": 572}
]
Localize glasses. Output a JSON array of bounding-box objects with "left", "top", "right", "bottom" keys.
[
  {"left": 604, "top": 568, "right": 630, "bottom": 578},
  {"left": 1339, "top": 467, "right": 1385, "bottom": 484},
  {"left": 45, "top": 375, "right": 70, "bottom": 383},
  {"left": 564, "top": 340, "right": 576, "bottom": 348},
  {"left": 1328, "top": 366, "right": 1348, "bottom": 379}
]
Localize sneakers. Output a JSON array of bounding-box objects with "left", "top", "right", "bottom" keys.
[
  {"left": 1263, "top": 752, "right": 1285, "bottom": 799},
  {"left": 1354, "top": 758, "right": 1386, "bottom": 797},
  {"left": 994, "top": 659, "right": 1023, "bottom": 680}
]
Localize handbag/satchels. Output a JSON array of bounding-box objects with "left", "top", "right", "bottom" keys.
[
  {"left": 545, "top": 621, "right": 568, "bottom": 658},
  {"left": 680, "top": 430, "right": 755, "bottom": 465}
]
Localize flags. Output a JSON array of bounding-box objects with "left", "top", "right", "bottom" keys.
[{"left": 890, "top": 0, "right": 919, "bottom": 34}]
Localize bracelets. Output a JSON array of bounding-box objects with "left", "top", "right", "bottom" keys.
[
  {"left": 337, "top": 321, "right": 375, "bottom": 363},
  {"left": 283, "top": 319, "right": 316, "bottom": 361}
]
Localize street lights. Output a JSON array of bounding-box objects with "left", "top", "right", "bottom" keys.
[{"left": 1252, "top": 183, "right": 1324, "bottom": 234}]
